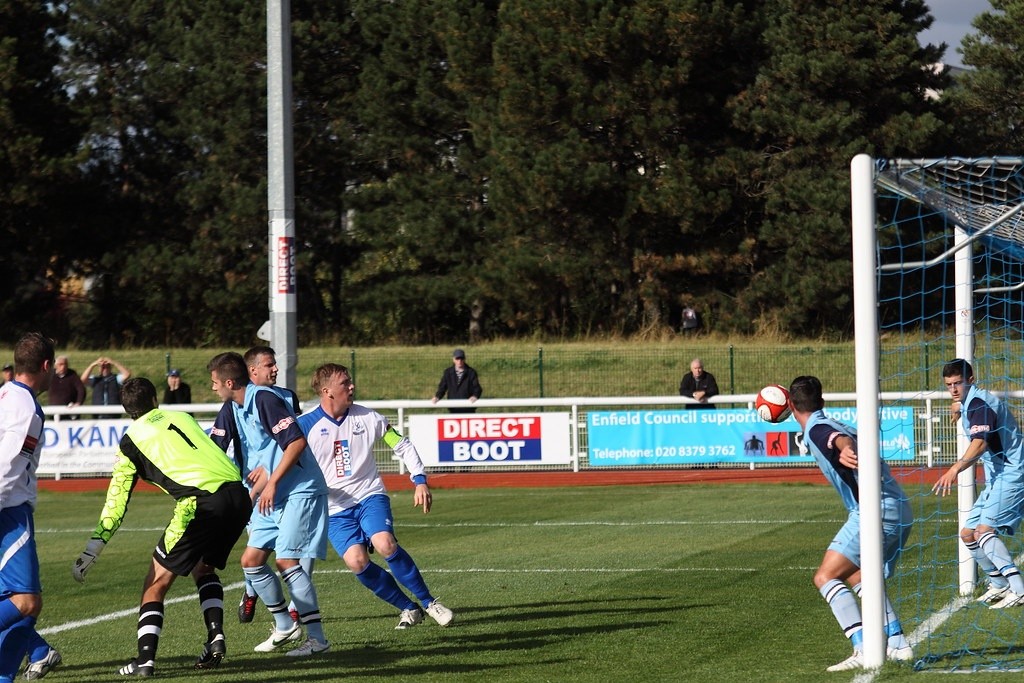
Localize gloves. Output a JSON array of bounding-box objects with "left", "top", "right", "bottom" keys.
[{"left": 73, "top": 539, "right": 106, "bottom": 582}]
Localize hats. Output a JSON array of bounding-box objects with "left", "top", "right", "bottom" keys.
[
  {"left": 454, "top": 349, "right": 465, "bottom": 359},
  {"left": 169, "top": 370, "right": 180, "bottom": 377}
]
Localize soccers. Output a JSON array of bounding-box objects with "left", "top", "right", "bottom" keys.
[{"left": 756, "top": 384, "right": 793, "bottom": 424}]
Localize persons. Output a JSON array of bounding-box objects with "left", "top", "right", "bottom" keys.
[
  {"left": 432, "top": 349, "right": 482, "bottom": 413},
  {"left": 777, "top": 375, "right": 915, "bottom": 671},
  {"left": 163, "top": 369, "right": 194, "bottom": 418},
  {"left": 44, "top": 356, "right": 86, "bottom": 420},
  {"left": 72, "top": 378, "right": 253, "bottom": 678},
  {"left": 0, "top": 331, "right": 62, "bottom": 683},
  {"left": 679, "top": 359, "right": 720, "bottom": 469},
  {"left": 206, "top": 348, "right": 332, "bottom": 657},
  {"left": 293, "top": 364, "right": 454, "bottom": 631},
  {"left": 931, "top": 359, "right": 1024, "bottom": 610},
  {"left": 80, "top": 356, "right": 131, "bottom": 419}
]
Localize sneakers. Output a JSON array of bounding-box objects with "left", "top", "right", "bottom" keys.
[
  {"left": 426, "top": 596, "right": 454, "bottom": 627},
  {"left": 988, "top": 592, "right": 1024, "bottom": 609},
  {"left": 286, "top": 638, "right": 330, "bottom": 656},
  {"left": 976, "top": 583, "right": 1010, "bottom": 602},
  {"left": 826, "top": 649, "right": 864, "bottom": 671},
  {"left": 886, "top": 646, "right": 914, "bottom": 661},
  {"left": 254, "top": 622, "right": 302, "bottom": 652},
  {"left": 289, "top": 611, "right": 299, "bottom": 623},
  {"left": 394, "top": 609, "right": 425, "bottom": 630},
  {"left": 114, "top": 657, "right": 154, "bottom": 677},
  {"left": 238, "top": 590, "right": 258, "bottom": 622},
  {"left": 194, "top": 634, "right": 226, "bottom": 671},
  {"left": 22, "top": 646, "right": 62, "bottom": 679}
]
